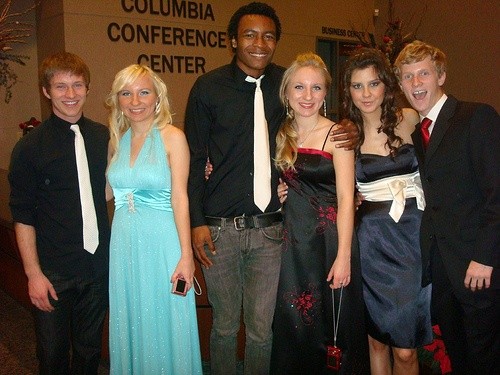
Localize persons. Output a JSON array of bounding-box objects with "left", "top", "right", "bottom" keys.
[
  {"left": 106, "top": 64, "right": 202, "bottom": 375},
  {"left": 355, "top": 40, "right": 500, "bottom": 375},
  {"left": 205, "top": 52, "right": 361, "bottom": 375},
  {"left": 277, "top": 49, "right": 433, "bottom": 375},
  {"left": 9, "top": 51, "right": 111, "bottom": 375},
  {"left": 183, "top": 3, "right": 361, "bottom": 375}
]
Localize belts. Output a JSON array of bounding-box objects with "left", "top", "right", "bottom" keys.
[{"left": 204, "top": 212, "right": 283, "bottom": 231}]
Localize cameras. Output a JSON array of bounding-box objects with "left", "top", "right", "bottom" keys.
[
  {"left": 171, "top": 278, "right": 188, "bottom": 296},
  {"left": 326, "top": 345, "right": 342, "bottom": 371}
]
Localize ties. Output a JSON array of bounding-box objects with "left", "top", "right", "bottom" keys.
[
  {"left": 70, "top": 124, "right": 99, "bottom": 255},
  {"left": 245, "top": 75, "right": 272, "bottom": 213},
  {"left": 419, "top": 118, "right": 432, "bottom": 153}
]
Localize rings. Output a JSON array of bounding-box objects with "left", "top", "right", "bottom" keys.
[{"left": 339, "top": 281, "right": 343, "bottom": 284}]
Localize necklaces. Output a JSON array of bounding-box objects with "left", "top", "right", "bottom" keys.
[{"left": 295, "top": 119, "right": 320, "bottom": 148}]
